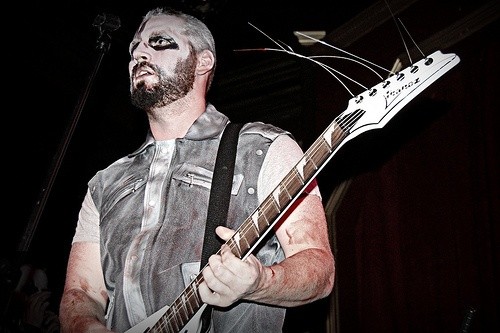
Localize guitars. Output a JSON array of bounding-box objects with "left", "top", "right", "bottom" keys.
[{"left": 122, "top": 50, "right": 460, "bottom": 333}]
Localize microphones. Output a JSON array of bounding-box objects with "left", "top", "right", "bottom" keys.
[{"left": 92, "top": 15, "right": 121, "bottom": 31}]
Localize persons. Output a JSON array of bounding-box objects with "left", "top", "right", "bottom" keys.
[
  {"left": 0, "top": 244, "right": 65, "bottom": 333},
  {"left": 57, "top": 6, "right": 336, "bottom": 333}
]
